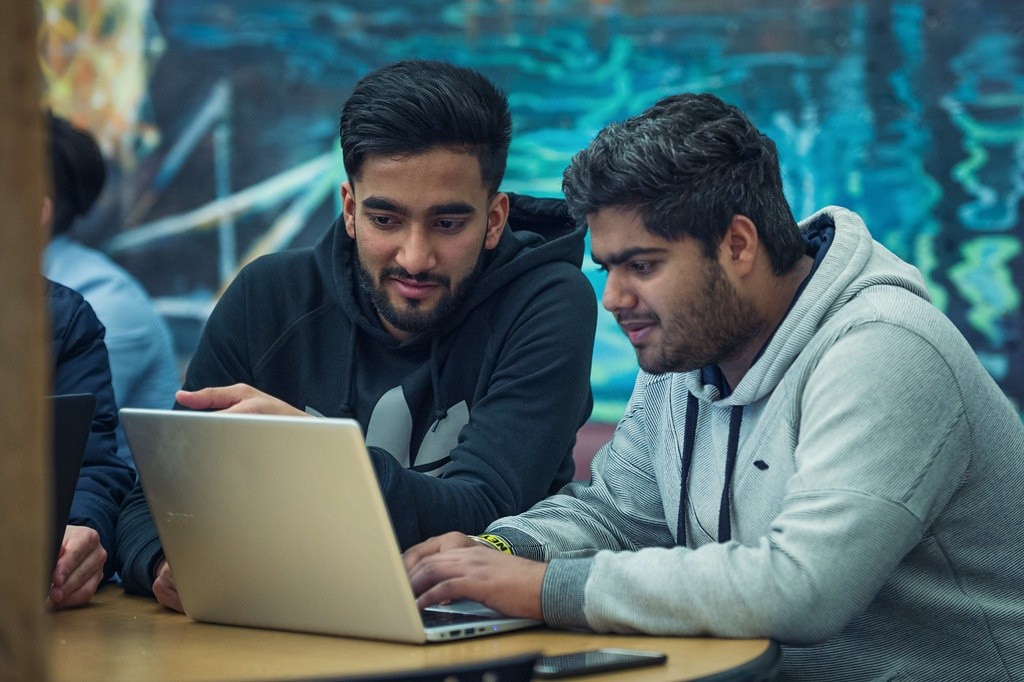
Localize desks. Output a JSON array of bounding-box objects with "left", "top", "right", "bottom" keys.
[{"left": 45, "top": 580, "right": 782, "bottom": 682}]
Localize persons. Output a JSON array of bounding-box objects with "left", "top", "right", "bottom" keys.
[
  {"left": 116, "top": 61, "right": 598, "bottom": 613},
  {"left": 37, "top": 110, "right": 183, "bottom": 612},
  {"left": 400, "top": 94, "right": 1023, "bottom": 681}
]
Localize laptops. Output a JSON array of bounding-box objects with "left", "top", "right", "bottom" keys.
[
  {"left": 117, "top": 408, "right": 542, "bottom": 643},
  {"left": 43, "top": 391, "right": 98, "bottom": 603}
]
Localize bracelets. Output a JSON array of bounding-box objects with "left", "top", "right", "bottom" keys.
[{"left": 468, "top": 534, "right": 513, "bottom": 557}]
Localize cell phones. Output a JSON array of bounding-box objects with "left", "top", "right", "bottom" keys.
[{"left": 530, "top": 648, "right": 666, "bottom": 679}]
